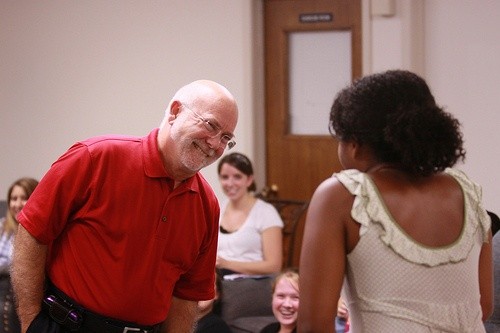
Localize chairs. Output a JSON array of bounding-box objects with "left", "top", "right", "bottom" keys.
[{"left": 195, "top": 200, "right": 309, "bottom": 333}]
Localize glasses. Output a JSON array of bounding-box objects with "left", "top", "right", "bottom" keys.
[{"left": 182, "top": 103, "right": 238, "bottom": 151}]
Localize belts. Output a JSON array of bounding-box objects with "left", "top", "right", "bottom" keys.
[{"left": 40, "top": 293, "right": 150, "bottom": 333}]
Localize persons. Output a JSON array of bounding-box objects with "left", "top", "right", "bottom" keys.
[
  {"left": 211, "top": 153, "right": 284, "bottom": 279},
  {"left": 12, "top": 80, "right": 239, "bottom": 333},
  {"left": 297, "top": 69, "right": 500, "bottom": 333},
  {"left": 259, "top": 267, "right": 300, "bottom": 333},
  {"left": 0, "top": 178, "right": 38, "bottom": 333}
]
